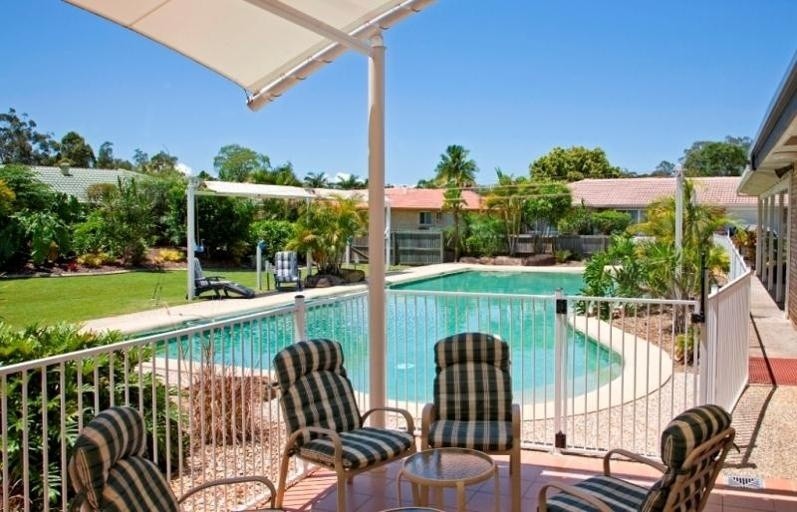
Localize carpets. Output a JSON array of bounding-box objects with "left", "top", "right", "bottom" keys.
[{"left": 746, "top": 356, "right": 796, "bottom": 388}]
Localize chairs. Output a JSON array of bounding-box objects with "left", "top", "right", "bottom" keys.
[
  {"left": 534, "top": 402, "right": 739, "bottom": 512},
  {"left": 64, "top": 405, "right": 286, "bottom": 512},
  {"left": 186, "top": 256, "right": 255, "bottom": 302},
  {"left": 268, "top": 250, "right": 303, "bottom": 292}
]
